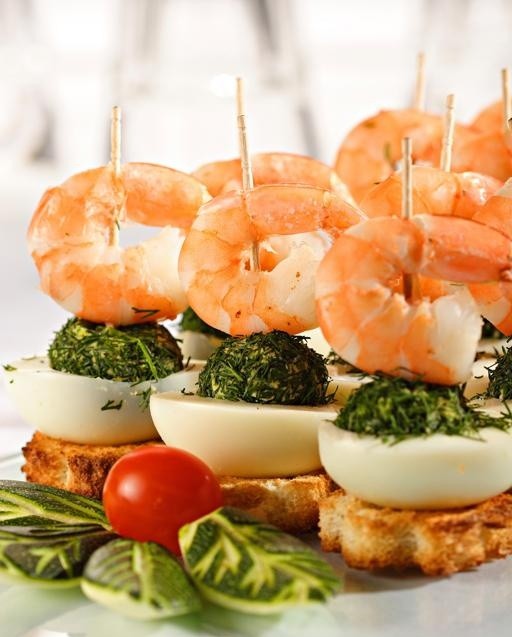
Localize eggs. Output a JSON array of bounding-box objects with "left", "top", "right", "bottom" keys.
[{"left": 3, "top": 327, "right": 512, "bottom": 511}]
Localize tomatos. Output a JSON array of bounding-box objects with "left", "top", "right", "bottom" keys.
[{"left": 103, "top": 448, "right": 225, "bottom": 558}]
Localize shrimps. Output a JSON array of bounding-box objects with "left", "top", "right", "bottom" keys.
[
  {"left": 188, "top": 153, "right": 357, "bottom": 209},
  {"left": 464, "top": 177, "right": 511, "bottom": 336},
  {"left": 27, "top": 163, "right": 214, "bottom": 327},
  {"left": 334, "top": 99, "right": 512, "bottom": 219},
  {"left": 313, "top": 213, "right": 511, "bottom": 385},
  {"left": 178, "top": 183, "right": 369, "bottom": 338}
]
